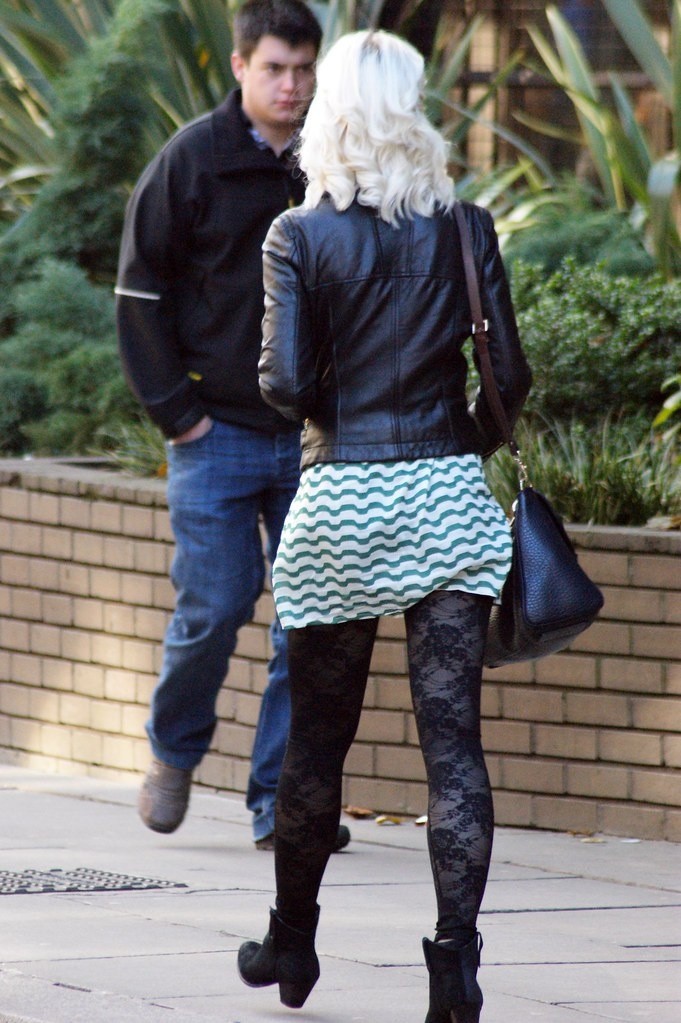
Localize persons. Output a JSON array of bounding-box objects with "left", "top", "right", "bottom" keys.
[
  {"left": 237, "top": 29, "right": 531, "bottom": 1023},
  {"left": 115, "top": 0, "right": 350, "bottom": 852}
]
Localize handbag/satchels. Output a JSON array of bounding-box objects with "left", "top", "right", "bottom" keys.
[{"left": 483, "top": 488, "right": 604, "bottom": 669}]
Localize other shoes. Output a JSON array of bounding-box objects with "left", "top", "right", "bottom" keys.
[
  {"left": 255, "top": 823, "right": 350, "bottom": 853},
  {"left": 138, "top": 753, "right": 192, "bottom": 834}
]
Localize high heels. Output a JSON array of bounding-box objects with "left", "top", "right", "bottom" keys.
[
  {"left": 421, "top": 932, "right": 484, "bottom": 1023},
  {"left": 238, "top": 905, "right": 320, "bottom": 1008}
]
